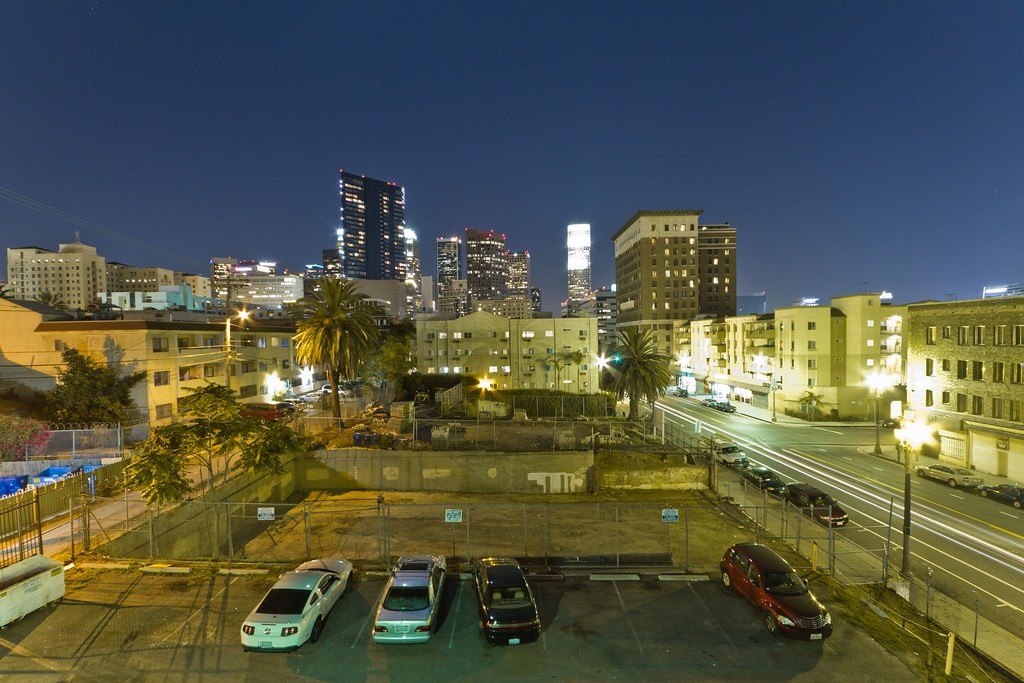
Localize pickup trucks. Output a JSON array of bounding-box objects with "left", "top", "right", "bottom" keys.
[{"left": 698, "top": 440, "right": 749, "bottom": 470}]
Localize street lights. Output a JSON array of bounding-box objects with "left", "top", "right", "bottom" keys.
[
  {"left": 890, "top": 421, "right": 937, "bottom": 579},
  {"left": 864, "top": 371, "right": 892, "bottom": 454},
  {"left": 770, "top": 375, "right": 779, "bottom": 422}
]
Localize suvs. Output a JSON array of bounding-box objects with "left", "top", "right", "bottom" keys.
[
  {"left": 300, "top": 392, "right": 323, "bottom": 402},
  {"left": 719, "top": 543, "right": 833, "bottom": 640},
  {"left": 284, "top": 399, "right": 308, "bottom": 414}
]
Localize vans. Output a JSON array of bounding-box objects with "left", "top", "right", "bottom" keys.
[
  {"left": 239, "top": 401, "right": 290, "bottom": 425},
  {"left": 322, "top": 384, "right": 353, "bottom": 397},
  {"left": 783, "top": 482, "right": 849, "bottom": 527}
]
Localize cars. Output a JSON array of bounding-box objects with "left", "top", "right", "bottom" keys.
[
  {"left": 473, "top": 556, "right": 542, "bottom": 647},
  {"left": 915, "top": 464, "right": 984, "bottom": 489},
  {"left": 672, "top": 386, "right": 688, "bottom": 397},
  {"left": 240, "top": 556, "right": 355, "bottom": 654},
  {"left": 372, "top": 553, "right": 449, "bottom": 646},
  {"left": 977, "top": 484, "right": 1024, "bottom": 510},
  {"left": 716, "top": 403, "right": 736, "bottom": 413},
  {"left": 701, "top": 399, "right": 718, "bottom": 408},
  {"left": 744, "top": 466, "right": 787, "bottom": 495}
]
